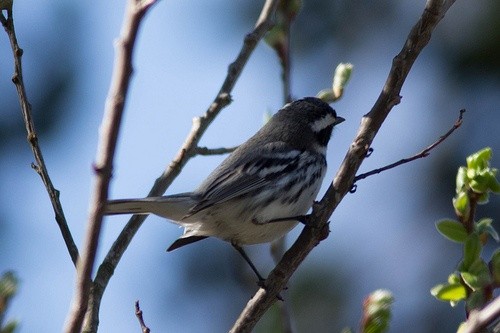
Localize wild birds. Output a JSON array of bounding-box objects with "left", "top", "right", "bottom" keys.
[{"left": 104, "top": 96, "right": 348, "bottom": 303}]
